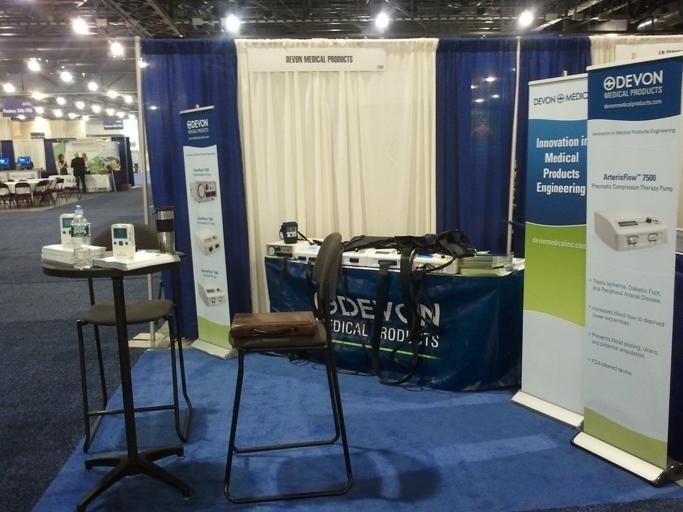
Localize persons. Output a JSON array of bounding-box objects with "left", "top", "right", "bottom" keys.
[
  {"left": 58, "top": 154, "right": 69, "bottom": 175},
  {"left": 71, "top": 152, "right": 90, "bottom": 193}
]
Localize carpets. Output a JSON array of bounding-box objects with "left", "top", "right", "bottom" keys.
[{"left": 28, "top": 322, "right": 680, "bottom": 510}]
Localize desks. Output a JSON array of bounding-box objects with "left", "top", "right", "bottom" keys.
[
  {"left": 262, "top": 246, "right": 524, "bottom": 391},
  {"left": 49, "top": 174, "right": 112, "bottom": 193},
  {"left": 42, "top": 248, "right": 202, "bottom": 509}
]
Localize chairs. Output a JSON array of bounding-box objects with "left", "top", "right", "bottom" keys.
[
  {"left": 223, "top": 233, "right": 355, "bottom": 504},
  {"left": 75, "top": 214, "right": 196, "bottom": 451},
  {"left": 0, "top": 178, "right": 64, "bottom": 210}
]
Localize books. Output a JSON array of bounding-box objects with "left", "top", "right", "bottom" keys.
[
  {"left": 459, "top": 251, "right": 517, "bottom": 277},
  {"left": 41, "top": 244, "right": 181, "bottom": 272}
]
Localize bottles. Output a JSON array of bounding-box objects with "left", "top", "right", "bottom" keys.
[
  {"left": 70, "top": 205, "right": 92, "bottom": 270},
  {"left": 153, "top": 206, "right": 175, "bottom": 256}
]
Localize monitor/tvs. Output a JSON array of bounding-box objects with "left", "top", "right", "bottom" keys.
[
  {"left": 17, "top": 156, "right": 31, "bottom": 171},
  {"left": 0, "top": 156, "right": 11, "bottom": 171}
]
